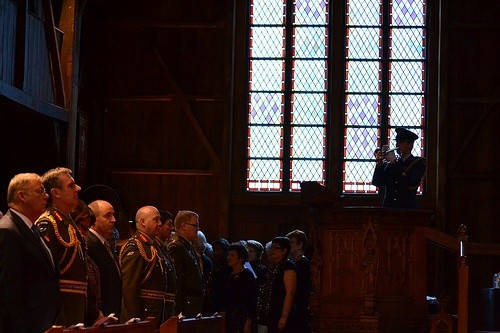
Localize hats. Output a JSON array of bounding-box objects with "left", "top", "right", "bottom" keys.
[{"left": 395, "top": 128, "right": 419, "bottom": 142}]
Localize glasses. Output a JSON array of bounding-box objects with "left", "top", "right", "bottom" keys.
[
  {"left": 28, "top": 189, "right": 46, "bottom": 196},
  {"left": 270, "top": 247, "right": 284, "bottom": 250},
  {"left": 78, "top": 222, "right": 88, "bottom": 234},
  {"left": 185, "top": 223, "right": 198, "bottom": 227}
]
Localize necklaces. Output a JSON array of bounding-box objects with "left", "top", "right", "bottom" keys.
[{"left": 295, "top": 254, "right": 304, "bottom": 260}]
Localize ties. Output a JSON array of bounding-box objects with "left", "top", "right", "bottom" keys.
[{"left": 32, "top": 225, "right": 54, "bottom": 272}]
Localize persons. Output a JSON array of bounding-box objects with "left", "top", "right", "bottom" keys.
[
  {"left": 0, "top": 166, "right": 309, "bottom": 333},
  {"left": 372, "top": 127, "right": 426, "bottom": 209}
]
